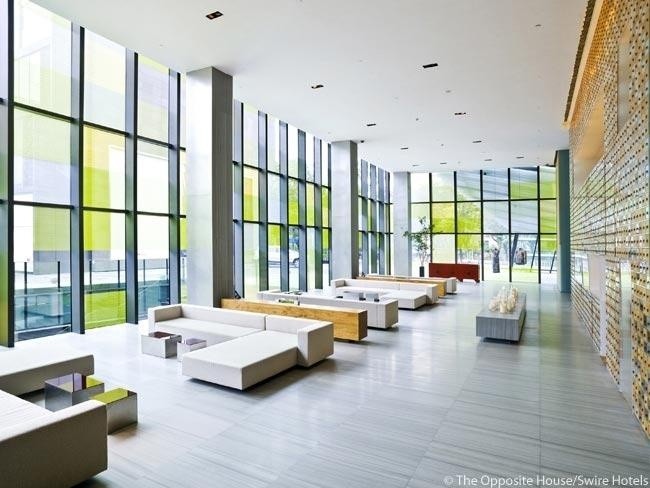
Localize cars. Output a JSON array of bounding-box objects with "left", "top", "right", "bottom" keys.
[{"left": 255, "top": 244, "right": 300, "bottom": 268}]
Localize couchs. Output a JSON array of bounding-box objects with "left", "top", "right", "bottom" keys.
[
  {"left": 1, "top": 332, "right": 108, "bottom": 488},
  {"left": 147, "top": 303, "right": 334, "bottom": 390},
  {"left": 257, "top": 272, "right": 457, "bottom": 330}
]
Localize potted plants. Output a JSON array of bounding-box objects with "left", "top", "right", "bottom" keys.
[{"left": 403, "top": 216, "right": 443, "bottom": 277}]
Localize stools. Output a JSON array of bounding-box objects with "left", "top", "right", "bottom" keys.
[{"left": 89, "top": 387, "right": 137, "bottom": 434}]
